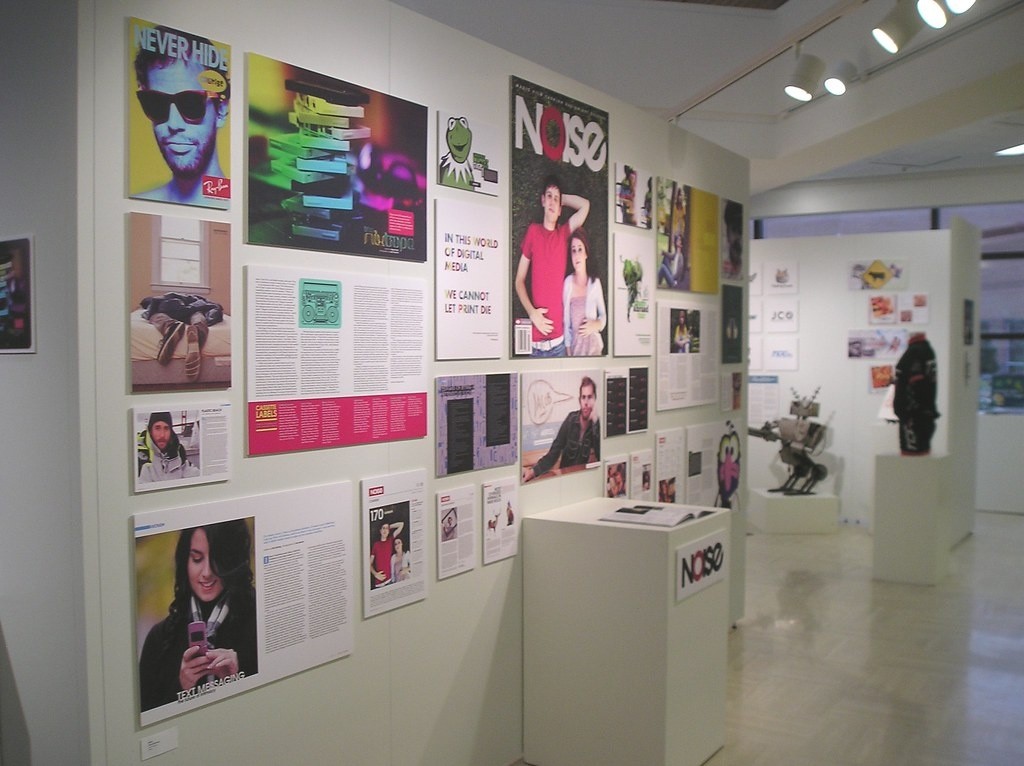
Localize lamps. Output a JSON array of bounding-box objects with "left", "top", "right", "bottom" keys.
[
  {"left": 872, "top": 0, "right": 929, "bottom": 55},
  {"left": 825, "top": 58, "right": 868, "bottom": 96},
  {"left": 917, "top": 0, "right": 953, "bottom": 30},
  {"left": 785, "top": 42, "right": 825, "bottom": 100}
]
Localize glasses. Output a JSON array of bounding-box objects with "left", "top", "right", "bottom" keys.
[{"left": 135, "top": 84, "right": 221, "bottom": 125}]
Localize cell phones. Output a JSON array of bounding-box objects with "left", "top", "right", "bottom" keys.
[{"left": 188, "top": 622, "right": 208, "bottom": 660}]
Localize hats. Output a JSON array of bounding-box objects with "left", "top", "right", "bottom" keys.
[{"left": 148, "top": 413, "right": 172, "bottom": 435}]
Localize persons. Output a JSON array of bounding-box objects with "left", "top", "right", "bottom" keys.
[
  {"left": 441, "top": 517, "right": 457, "bottom": 541},
  {"left": 673, "top": 311, "right": 691, "bottom": 354},
  {"left": 619, "top": 165, "right": 638, "bottom": 227},
  {"left": 139, "top": 519, "right": 258, "bottom": 712},
  {"left": 608, "top": 465, "right": 625, "bottom": 497},
  {"left": 523, "top": 377, "right": 600, "bottom": 482},
  {"left": 130, "top": 25, "right": 229, "bottom": 209},
  {"left": 891, "top": 331, "right": 943, "bottom": 456},
  {"left": 137, "top": 412, "right": 200, "bottom": 484},
  {"left": 658, "top": 235, "right": 684, "bottom": 289},
  {"left": 369, "top": 519, "right": 411, "bottom": 589},
  {"left": 150, "top": 312, "right": 209, "bottom": 381},
  {"left": 515, "top": 175, "right": 607, "bottom": 359}
]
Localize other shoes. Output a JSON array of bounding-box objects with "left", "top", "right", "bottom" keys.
[
  {"left": 184, "top": 327, "right": 202, "bottom": 383},
  {"left": 157, "top": 320, "right": 185, "bottom": 366}
]
